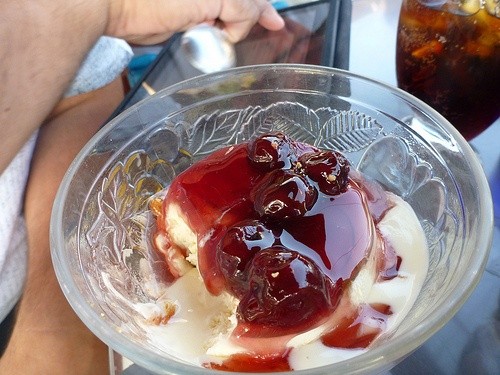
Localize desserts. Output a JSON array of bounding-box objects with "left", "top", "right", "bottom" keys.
[{"left": 139, "top": 131, "right": 431, "bottom": 372}]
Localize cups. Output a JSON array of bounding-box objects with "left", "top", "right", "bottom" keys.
[{"left": 396, "top": 0, "right": 500, "bottom": 142}]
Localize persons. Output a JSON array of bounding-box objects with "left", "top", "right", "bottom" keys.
[
  {"left": 0, "top": 0, "right": 287, "bottom": 177},
  {"left": 0, "top": 35, "right": 134, "bottom": 374}
]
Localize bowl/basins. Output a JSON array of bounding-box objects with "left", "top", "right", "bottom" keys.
[{"left": 49, "top": 61, "right": 494, "bottom": 375}]
